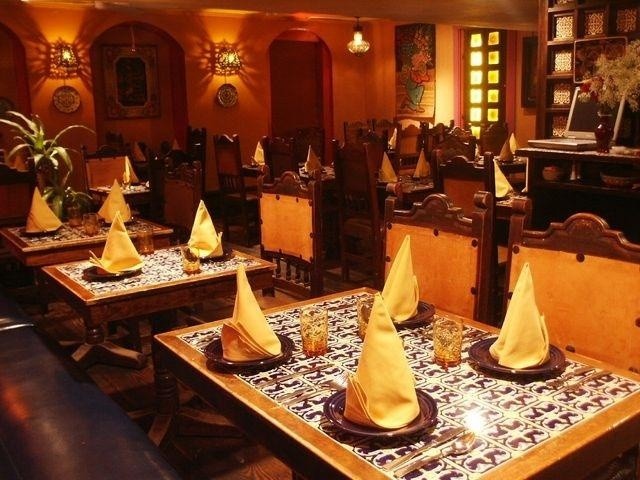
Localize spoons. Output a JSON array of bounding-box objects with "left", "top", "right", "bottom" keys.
[{"left": 392, "top": 432, "right": 478, "bottom": 478}]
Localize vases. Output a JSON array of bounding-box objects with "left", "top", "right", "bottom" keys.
[{"left": 593, "top": 108, "right": 616, "bottom": 153}]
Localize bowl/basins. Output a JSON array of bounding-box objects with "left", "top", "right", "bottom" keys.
[
  {"left": 541, "top": 167, "right": 568, "bottom": 182},
  {"left": 598, "top": 168, "right": 640, "bottom": 187}
]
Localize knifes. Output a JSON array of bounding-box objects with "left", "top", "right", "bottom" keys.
[
  {"left": 545, "top": 366, "right": 612, "bottom": 390},
  {"left": 254, "top": 363, "right": 335, "bottom": 389},
  {"left": 382, "top": 426, "right": 466, "bottom": 472}
]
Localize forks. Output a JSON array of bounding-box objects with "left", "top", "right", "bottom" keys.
[
  {"left": 276, "top": 370, "right": 350, "bottom": 406},
  {"left": 404, "top": 326, "right": 432, "bottom": 337}
]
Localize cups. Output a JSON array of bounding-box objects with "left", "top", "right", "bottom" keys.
[
  {"left": 356, "top": 296, "right": 384, "bottom": 341},
  {"left": 83, "top": 212, "right": 99, "bottom": 236},
  {"left": 135, "top": 223, "right": 154, "bottom": 254},
  {"left": 121, "top": 180, "right": 131, "bottom": 191},
  {"left": 300, "top": 306, "right": 329, "bottom": 355},
  {"left": 432, "top": 315, "right": 463, "bottom": 367}
]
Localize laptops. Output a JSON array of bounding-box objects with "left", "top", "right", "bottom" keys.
[{"left": 527, "top": 87, "right": 625, "bottom": 152}]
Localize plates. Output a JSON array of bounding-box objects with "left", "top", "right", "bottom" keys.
[
  {"left": 17, "top": 226, "right": 59, "bottom": 235},
  {"left": 324, "top": 388, "right": 438, "bottom": 437},
  {"left": 204, "top": 333, "right": 294, "bottom": 367},
  {"left": 180, "top": 244, "right": 200, "bottom": 272},
  {"left": 198, "top": 248, "right": 232, "bottom": 261},
  {"left": 362, "top": 300, "right": 436, "bottom": 325},
  {"left": 468, "top": 337, "right": 567, "bottom": 376},
  {"left": 84, "top": 264, "right": 141, "bottom": 279},
  {"left": 99, "top": 216, "right": 138, "bottom": 227}
]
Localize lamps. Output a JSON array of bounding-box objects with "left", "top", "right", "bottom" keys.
[
  {"left": 345, "top": 15, "right": 373, "bottom": 57},
  {"left": 48, "top": 42, "right": 81, "bottom": 75},
  {"left": 210, "top": 43, "right": 246, "bottom": 77}
]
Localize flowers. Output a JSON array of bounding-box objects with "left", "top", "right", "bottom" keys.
[{"left": 579, "top": 43, "right": 639, "bottom": 108}]
